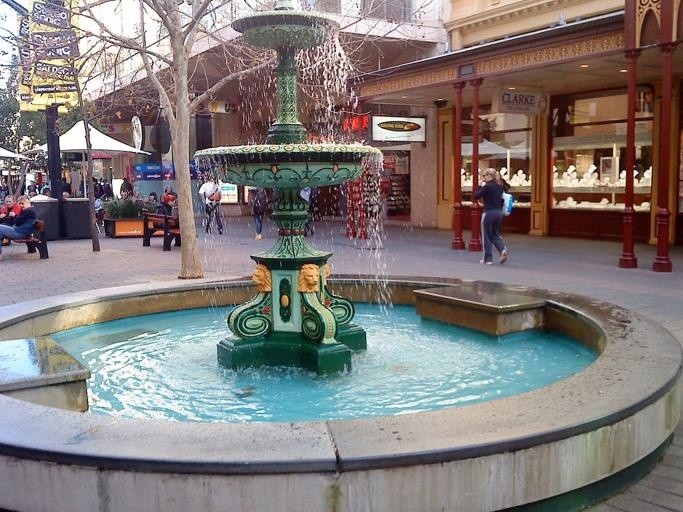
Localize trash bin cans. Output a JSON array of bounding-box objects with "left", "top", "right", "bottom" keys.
[{"left": 29, "top": 197, "right": 95, "bottom": 241}]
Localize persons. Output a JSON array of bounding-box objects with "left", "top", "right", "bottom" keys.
[
  {"left": 198, "top": 179, "right": 223, "bottom": 235},
  {"left": 38, "top": 177, "right": 71, "bottom": 198},
  {"left": 247, "top": 185, "right": 269, "bottom": 240},
  {"left": 304, "top": 187, "right": 321, "bottom": 236},
  {"left": 496, "top": 170, "right": 511, "bottom": 239},
  {"left": 0, "top": 194, "right": 38, "bottom": 261},
  {"left": 474, "top": 167, "right": 510, "bottom": 265},
  {"left": 0, "top": 194, "right": 21, "bottom": 242},
  {"left": 27, "top": 180, "right": 37, "bottom": 197},
  {"left": 148, "top": 185, "right": 181, "bottom": 247},
  {"left": 79, "top": 176, "right": 134, "bottom": 198}
]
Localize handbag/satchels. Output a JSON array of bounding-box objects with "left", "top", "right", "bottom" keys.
[
  {"left": 209, "top": 191, "right": 221, "bottom": 201},
  {"left": 501, "top": 191, "right": 513, "bottom": 216}
]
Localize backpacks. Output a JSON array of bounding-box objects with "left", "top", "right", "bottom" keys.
[{"left": 254, "top": 197, "right": 266, "bottom": 215}]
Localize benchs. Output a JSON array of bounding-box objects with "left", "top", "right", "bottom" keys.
[
  {"left": 140, "top": 209, "right": 180, "bottom": 252},
  {"left": 1, "top": 219, "right": 48, "bottom": 260}
]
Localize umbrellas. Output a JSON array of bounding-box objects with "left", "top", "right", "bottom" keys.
[
  {"left": 21, "top": 120, "right": 152, "bottom": 198},
  {"left": 0, "top": 147, "right": 32, "bottom": 161}
]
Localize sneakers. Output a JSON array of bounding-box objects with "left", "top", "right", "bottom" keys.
[
  {"left": 499, "top": 250, "right": 508, "bottom": 264},
  {"left": 219, "top": 230, "right": 223, "bottom": 234},
  {"left": 480, "top": 260, "right": 492, "bottom": 264},
  {"left": 255, "top": 234, "right": 262, "bottom": 240}
]
